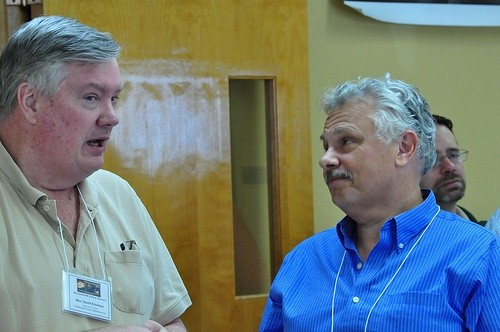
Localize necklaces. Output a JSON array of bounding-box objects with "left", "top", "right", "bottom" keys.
[{"left": 330, "top": 204, "right": 440, "bottom": 332}]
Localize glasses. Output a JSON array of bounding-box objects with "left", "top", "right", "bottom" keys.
[{"left": 433, "top": 149, "right": 469, "bottom": 168}]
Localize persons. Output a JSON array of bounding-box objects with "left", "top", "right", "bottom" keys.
[
  {"left": 0, "top": 15, "right": 194, "bottom": 332},
  {"left": 419, "top": 114, "right": 488, "bottom": 227},
  {"left": 257, "top": 72, "right": 500, "bottom": 332}
]
